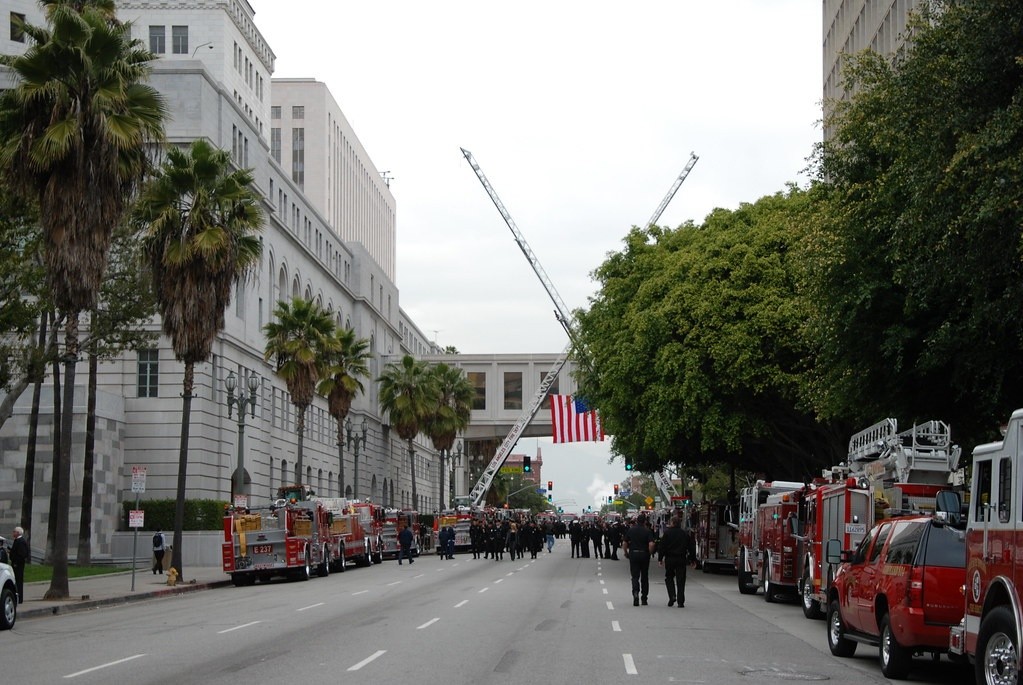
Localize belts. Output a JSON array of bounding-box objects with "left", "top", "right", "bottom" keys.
[{"left": 632, "top": 550, "right": 646, "bottom": 552}]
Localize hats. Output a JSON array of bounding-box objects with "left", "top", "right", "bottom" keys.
[
  {"left": 613, "top": 521, "right": 619, "bottom": 526},
  {"left": 573, "top": 520, "right": 579, "bottom": 524}
]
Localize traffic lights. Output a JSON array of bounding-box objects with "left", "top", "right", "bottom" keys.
[
  {"left": 558, "top": 507, "right": 561, "bottom": 513},
  {"left": 625, "top": 455, "right": 632, "bottom": 471},
  {"left": 588, "top": 506, "right": 591, "bottom": 511},
  {"left": 609, "top": 496, "right": 612, "bottom": 504},
  {"left": 685, "top": 490, "right": 692, "bottom": 504},
  {"left": 548, "top": 495, "right": 552, "bottom": 502},
  {"left": 548, "top": 481, "right": 552, "bottom": 490},
  {"left": 523, "top": 456, "right": 530, "bottom": 472},
  {"left": 614, "top": 484, "right": 619, "bottom": 494}
]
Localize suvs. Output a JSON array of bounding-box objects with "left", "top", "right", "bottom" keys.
[{"left": 824, "top": 514, "right": 971, "bottom": 679}]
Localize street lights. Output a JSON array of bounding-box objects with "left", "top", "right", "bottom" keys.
[
  {"left": 345, "top": 417, "right": 369, "bottom": 500},
  {"left": 444, "top": 439, "right": 462, "bottom": 510},
  {"left": 224, "top": 367, "right": 260, "bottom": 493}
]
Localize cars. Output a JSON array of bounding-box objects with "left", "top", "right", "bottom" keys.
[{"left": 0, "top": 560, "right": 18, "bottom": 630}]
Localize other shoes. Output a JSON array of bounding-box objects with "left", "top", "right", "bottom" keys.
[
  {"left": 634, "top": 597, "right": 639, "bottom": 606},
  {"left": 472, "top": 552, "right": 622, "bottom": 562},
  {"left": 678, "top": 601, "right": 684, "bottom": 607},
  {"left": 399, "top": 560, "right": 402, "bottom": 565},
  {"left": 641, "top": 597, "right": 647, "bottom": 605},
  {"left": 410, "top": 560, "right": 414, "bottom": 564},
  {"left": 152, "top": 568, "right": 157, "bottom": 574},
  {"left": 159, "top": 570, "right": 163, "bottom": 574},
  {"left": 668, "top": 599, "right": 676, "bottom": 606}
]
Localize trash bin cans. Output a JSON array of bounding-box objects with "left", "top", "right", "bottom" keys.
[{"left": 152, "top": 544, "right": 173, "bottom": 570}]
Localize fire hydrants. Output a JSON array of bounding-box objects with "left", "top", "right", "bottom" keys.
[{"left": 165, "top": 568, "right": 178, "bottom": 586}]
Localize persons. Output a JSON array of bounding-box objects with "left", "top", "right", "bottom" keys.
[
  {"left": 7, "top": 526, "right": 28, "bottom": 604},
  {"left": 152, "top": 528, "right": 165, "bottom": 574},
  {"left": 568, "top": 520, "right": 653, "bottom": 561},
  {"left": 0, "top": 537, "right": 8, "bottom": 564},
  {"left": 469, "top": 518, "right": 567, "bottom": 561},
  {"left": 398, "top": 526, "right": 414, "bottom": 565},
  {"left": 657, "top": 517, "right": 698, "bottom": 608},
  {"left": 439, "top": 526, "right": 456, "bottom": 560},
  {"left": 623, "top": 515, "right": 655, "bottom": 607}
]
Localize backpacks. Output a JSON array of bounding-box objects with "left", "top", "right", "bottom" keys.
[{"left": 153, "top": 533, "right": 162, "bottom": 547}]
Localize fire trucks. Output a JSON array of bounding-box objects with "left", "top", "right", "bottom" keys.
[
  {"left": 221, "top": 483, "right": 416, "bottom": 584},
  {"left": 535, "top": 510, "right": 655, "bottom": 533},
  {"left": 725, "top": 479, "right": 812, "bottom": 604},
  {"left": 931, "top": 404, "right": 1023, "bottom": 685},
  {"left": 786, "top": 412, "right": 966, "bottom": 620},
  {"left": 456, "top": 146, "right": 744, "bottom": 575},
  {"left": 432, "top": 152, "right": 700, "bottom": 552}
]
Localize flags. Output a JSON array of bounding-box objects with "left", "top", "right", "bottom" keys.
[{"left": 551, "top": 394, "right": 604, "bottom": 444}]
